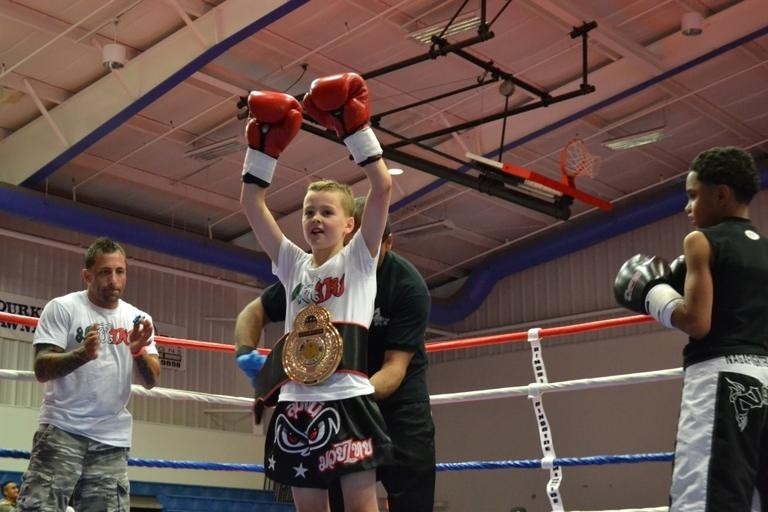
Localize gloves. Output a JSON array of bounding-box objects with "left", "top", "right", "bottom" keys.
[
  {"left": 614, "top": 253, "right": 687, "bottom": 330},
  {"left": 301, "top": 73, "right": 381, "bottom": 166},
  {"left": 236, "top": 350, "right": 267, "bottom": 388},
  {"left": 240, "top": 90, "right": 301, "bottom": 187}
]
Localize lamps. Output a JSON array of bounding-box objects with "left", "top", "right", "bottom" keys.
[
  {"left": 101, "top": 20, "right": 126, "bottom": 70},
  {"left": 680, "top": 12, "right": 703, "bottom": 36}
]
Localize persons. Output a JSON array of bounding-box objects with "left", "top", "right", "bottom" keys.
[
  {"left": 17, "top": 238, "right": 163, "bottom": 511},
  {"left": 238, "top": 69, "right": 399, "bottom": 512},
  {"left": 234, "top": 199, "right": 438, "bottom": 511},
  {"left": 610, "top": 147, "right": 767, "bottom": 511},
  {"left": 0, "top": 481, "right": 20, "bottom": 512}
]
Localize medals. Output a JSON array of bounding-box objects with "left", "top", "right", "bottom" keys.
[{"left": 282, "top": 301, "right": 344, "bottom": 386}]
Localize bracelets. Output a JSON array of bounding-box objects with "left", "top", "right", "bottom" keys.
[{"left": 132, "top": 347, "right": 144, "bottom": 358}]
